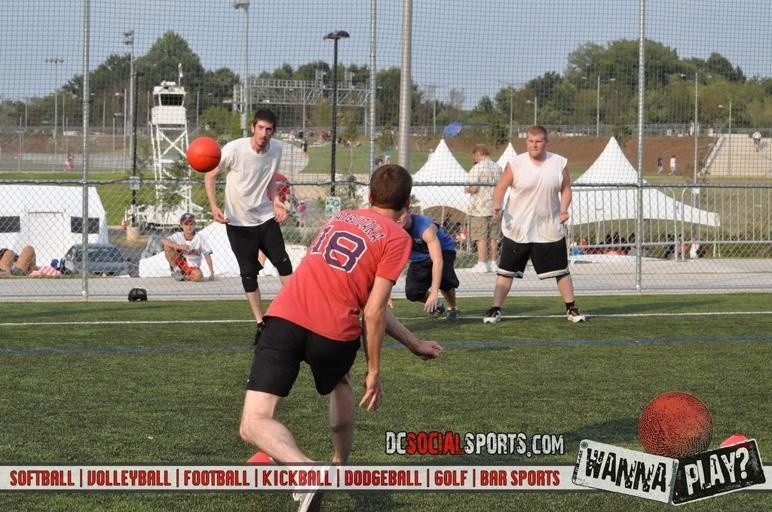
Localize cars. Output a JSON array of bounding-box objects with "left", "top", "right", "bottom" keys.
[
  {"left": 278, "top": 214, "right": 325, "bottom": 249},
  {"left": 61, "top": 243, "right": 131, "bottom": 278}
]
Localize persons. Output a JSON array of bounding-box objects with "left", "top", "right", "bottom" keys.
[
  {"left": 394, "top": 203, "right": 463, "bottom": 324},
  {"left": 467, "top": 145, "right": 503, "bottom": 273},
  {"left": 162, "top": 212, "right": 216, "bottom": 282},
  {"left": 656, "top": 157, "right": 664, "bottom": 176},
  {"left": 1, "top": 245, "right": 37, "bottom": 277},
  {"left": 432, "top": 212, "right": 707, "bottom": 260},
  {"left": 347, "top": 172, "right": 357, "bottom": 199},
  {"left": 65, "top": 151, "right": 75, "bottom": 172},
  {"left": 751, "top": 129, "right": 761, "bottom": 152},
  {"left": 202, "top": 106, "right": 295, "bottom": 346},
  {"left": 297, "top": 119, "right": 713, "bottom": 169},
  {"left": 295, "top": 198, "right": 307, "bottom": 227},
  {"left": 238, "top": 163, "right": 445, "bottom": 512},
  {"left": 668, "top": 154, "right": 677, "bottom": 176},
  {"left": 483, "top": 125, "right": 587, "bottom": 325}
]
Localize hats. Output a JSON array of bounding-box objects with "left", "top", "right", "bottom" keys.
[{"left": 180, "top": 213, "right": 196, "bottom": 223}]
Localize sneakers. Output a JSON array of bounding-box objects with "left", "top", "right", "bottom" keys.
[
  {"left": 465, "top": 262, "right": 487, "bottom": 273},
  {"left": 291, "top": 459, "right": 336, "bottom": 512},
  {"left": 482, "top": 306, "right": 501, "bottom": 324},
  {"left": 171, "top": 269, "right": 186, "bottom": 282},
  {"left": 486, "top": 262, "right": 498, "bottom": 272},
  {"left": 444, "top": 307, "right": 459, "bottom": 321},
  {"left": 431, "top": 303, "right": 445, "bottom": 319},
  {"left": 565, "top": 306, "right": 586, "bottom": 323}
]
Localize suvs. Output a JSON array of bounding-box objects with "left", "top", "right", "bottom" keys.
[{"left": 137, "top": 228, "right": 199, "bottom": 260}]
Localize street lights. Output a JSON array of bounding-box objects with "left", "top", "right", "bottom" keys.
[
  {"left": 717, "top": 100, "right": 732, "bottom": 177},
  {"left": 580, "top": 73, "right": 616, "bottom": 136},
  {"left": 526, "top": 97, "right": 537, "bottom": 128},
  {"left": 495, "top": 77, "right": 529, "bottom": 141},
  {"left": 288, "top": 67, "right": 382, "bottom": 141},
  {"left": 232, "top": 0, "right": 253, "bottom": 138},
  {"left": 420, "top": 81, "right": 451, "bottom": 135},
  {"left": 680, "top": 72, "right": 714, "bottom": 185},
  {"left": 12, "top": 28, "right": 135, "bottom": 176},
  {"left": 323, "top": 30, "right": 351, "bottom": 199}
]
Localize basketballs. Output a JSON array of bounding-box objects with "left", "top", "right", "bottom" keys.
[
  {"left": 638, "top": 393, "right": 712, "bottom": 462},
  {"left": 188, "top": 138, "right": 222, "bottom": 172}
]
